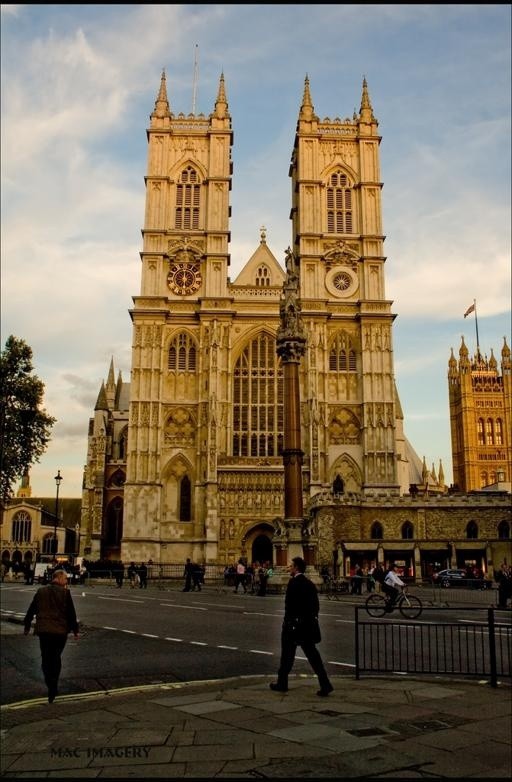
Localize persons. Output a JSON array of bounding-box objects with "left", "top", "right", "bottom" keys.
[
  {"left": 269, "top": 556, "right": 334, "bottom": 696},
  {"left": 381, "top": 564, "right": 407, "bottom": 613},
  {"left": 495, "top": 563, "right": 512, "bottom": 608},
  {"left": 114, "top": 560, "right": 148, "bottom": 589},
  {"left": 181, "top": 556, "right": 273, "bottom": 596},
  {"left": 349, "top": 563, "right": 384, "bottom": 595},
  {"left": 23, "top": 568, "right": 80, "bottom": 706}
]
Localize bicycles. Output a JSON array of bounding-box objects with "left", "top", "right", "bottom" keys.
[{"left": 363, "top": 583, "right": 425, "bottom": 620}]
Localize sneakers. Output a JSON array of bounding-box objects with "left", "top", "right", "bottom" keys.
[
  {"left": 270, "top": 680, "right": 289, "bottom": 692},
  {"left": 317, "top": 687, "right": 333, "bottom": 697}
]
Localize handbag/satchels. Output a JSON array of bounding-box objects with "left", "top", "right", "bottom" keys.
[{"left": 293, "top": 617, "right": 323, "bottom": 646}]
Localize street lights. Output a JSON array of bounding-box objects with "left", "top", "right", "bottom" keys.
[{"left": 50, "top": 467, "right": 63, "bottom": 561}]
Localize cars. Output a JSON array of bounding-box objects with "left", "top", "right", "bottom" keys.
[{"left": 430, "top": 567, "right": 494, "bottom": 591}]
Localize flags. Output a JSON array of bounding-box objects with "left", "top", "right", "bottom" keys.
[{"left": 464, "top": 304, "right": 475, "bottom": 318}]
